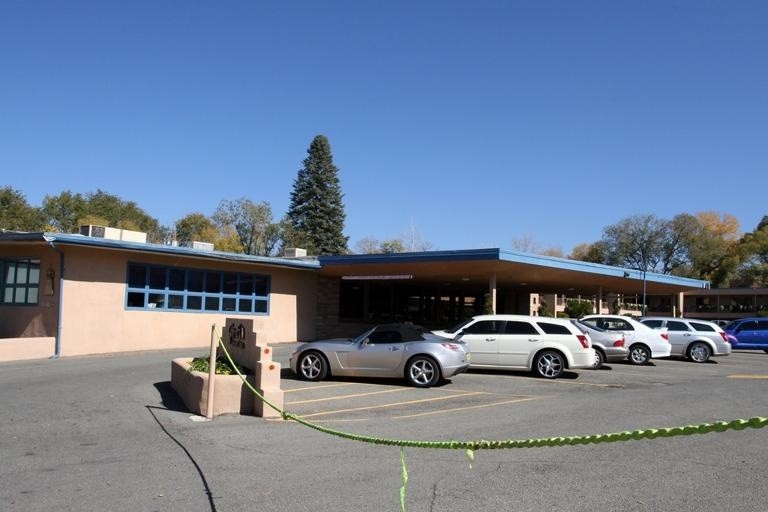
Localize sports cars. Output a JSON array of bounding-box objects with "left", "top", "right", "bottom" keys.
[{"left": 290, "top": 324, "right": 474, "bottom": 387}]
